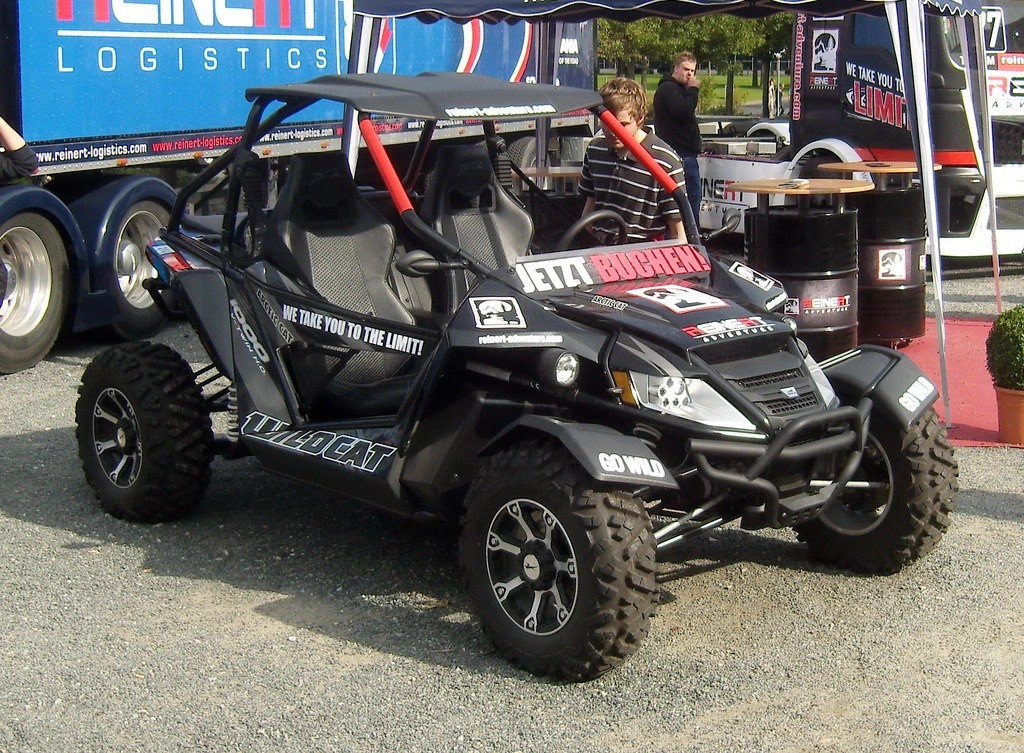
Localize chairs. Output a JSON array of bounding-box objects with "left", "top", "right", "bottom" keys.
[
  {"left": 260, "top": 149, "right": 421, "bottom": 417},
  {"left": 422, "top": 144, "right": 536, "bottom": 318}
]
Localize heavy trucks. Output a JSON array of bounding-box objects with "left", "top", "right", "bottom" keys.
[
  {"left": 644, "top": 1, "right": 1023, "bottom": 262},
  {"left": 1, "top": 1, "right": 607, "bottom": 372}
]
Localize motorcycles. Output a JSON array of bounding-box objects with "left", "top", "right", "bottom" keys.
[{"left": 72, "top": 63, "right": 963, "bottom": 685}]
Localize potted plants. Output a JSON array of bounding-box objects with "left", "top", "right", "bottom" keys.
[{"left": 985, "top": 302, "right": 1024, "bottom": 445}]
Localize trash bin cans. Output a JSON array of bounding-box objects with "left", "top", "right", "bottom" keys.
[{"left": 724, "top": 151, "right": 933, "bottom": 357}]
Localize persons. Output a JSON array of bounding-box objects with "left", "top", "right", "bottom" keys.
[
  {"left": 0, "top": 116, "right": 39, "bottom": 182},
  {"left": 776, "top": 83, "right": 791, "bottom": 116},
  {"left": 577, "top": 78, "right": 688, "bottom": 247},
  {"left": 654, "top": 51, "right": 703, "bottom": 245}
]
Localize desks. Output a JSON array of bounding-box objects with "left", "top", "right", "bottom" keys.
[
  {"left": 726, "top": 179, "right": 875, "bottom": 214},
  {"left": 816, "top": 161, "right": 942, "bottom": 191}
]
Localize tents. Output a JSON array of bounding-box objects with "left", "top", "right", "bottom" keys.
[{"left": 342, "top": 0, "right": 1001, "bottom": 429}]
